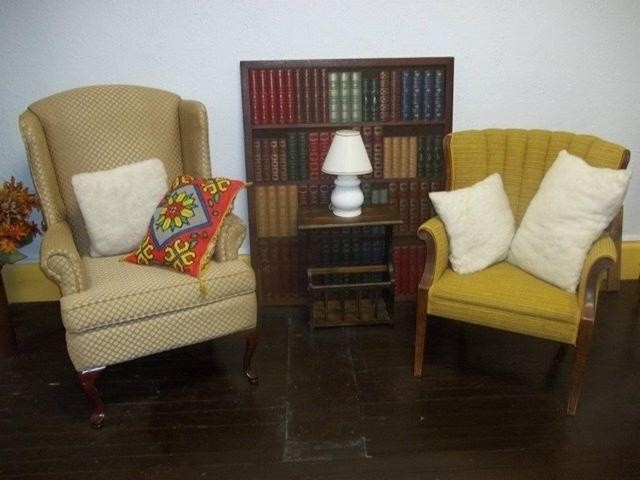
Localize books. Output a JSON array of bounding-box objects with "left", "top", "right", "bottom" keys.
[
  {"left": 253, "top": 181, "right": 446, "bottom": 237},
  {"left": 259, "top": 226, "right": 388, "bottom": 297},
  {"left": 252, "top": 126, "right": 447, "bottom": 181},
  {"left": 391, "top": 237, "right": 427, "bottom": 296},
  {"left": 249, "top": 66, "right": 447, "bottom": 126}
]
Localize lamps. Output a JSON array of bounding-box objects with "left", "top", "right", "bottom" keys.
[{"left": 321, "top": 130, "right": 373, "bottom": 218}]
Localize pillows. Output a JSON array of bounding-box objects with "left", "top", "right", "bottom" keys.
[
  {"left": 506, "top": 150, "right": 632, "bottom": 295},
  {"left": 428, "top": 172, "right": 515, "bottom": 275},
  {"left": 71, "top": 158, "right": 169, "bottom": 258},
  {"left": 119, "top": 174, "right": 253, "bottom": 296}
]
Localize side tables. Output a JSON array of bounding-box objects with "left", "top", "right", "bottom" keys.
[{"left": 296, "top": 204, "right": 404, "bottom": 333}]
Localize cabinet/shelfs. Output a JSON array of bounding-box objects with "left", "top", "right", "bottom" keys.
[{"left": 240, "top": 57, "right": 454, "bottom": 307}]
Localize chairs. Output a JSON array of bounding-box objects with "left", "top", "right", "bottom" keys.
[
  {"left": 18, "top": 85, "right": 258, "bottom": 430},
  {"left": 414, "top": 128, "right": 634, "bottom": 416}
]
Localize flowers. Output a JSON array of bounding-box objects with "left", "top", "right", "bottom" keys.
[{"left": 0, "top": 176, "right": 48, "bottom": 264}]
